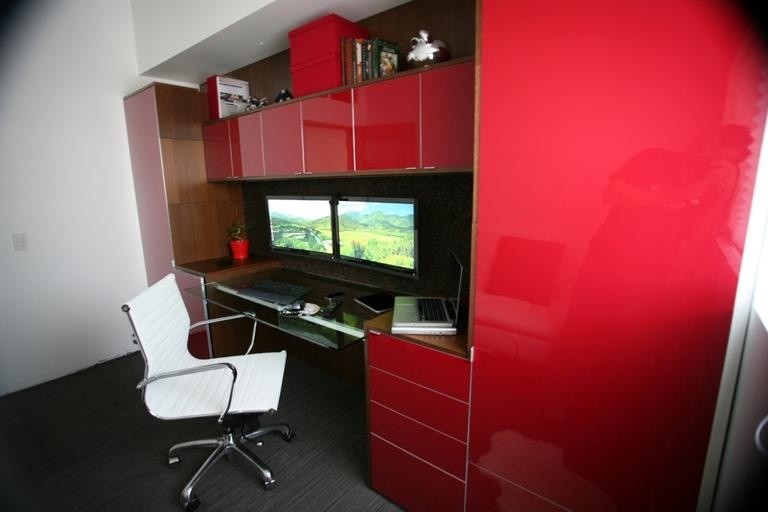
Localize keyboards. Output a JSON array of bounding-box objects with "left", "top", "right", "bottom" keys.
[{"left": 239, "top": 279, "right": 312, "bottom": 313}]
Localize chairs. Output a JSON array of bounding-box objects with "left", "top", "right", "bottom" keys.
[{"left": 121, "top": 273, "right": 295, "bottom": 511}]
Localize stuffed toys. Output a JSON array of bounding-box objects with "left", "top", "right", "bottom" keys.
[{"left": 244, "top": 88, "right": 293, "bottom": 112}]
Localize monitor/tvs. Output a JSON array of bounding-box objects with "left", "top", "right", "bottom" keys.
[
  {"left": 264, "top": 191, "right": 335, "bottom": 281},
  {"left": 335, "top": 193, "right": 420, "bottom": 287}
]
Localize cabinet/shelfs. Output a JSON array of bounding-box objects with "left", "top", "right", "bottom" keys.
[
  {"left": 362, "top": 324, "right": 471, "bottom": 512},
  {"left": 123, "top": 80, "right": 244, "bottom": 359},
  {"left": 202, "top": 57, "right": 476, "bottom": 183},
  {"left": 466, "top": 0, "right": 768, "bottom": 512},
  {"left": 174, "top": 264, "right": 282, "bottom": 359}
]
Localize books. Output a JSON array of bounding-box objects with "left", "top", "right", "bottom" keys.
[{"left": 340, "top": 34, "right": 402, "bottom": 87}]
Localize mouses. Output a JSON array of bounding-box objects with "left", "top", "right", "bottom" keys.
[{"left": 293, "top": 299, "right": 305, "bottom": 312}]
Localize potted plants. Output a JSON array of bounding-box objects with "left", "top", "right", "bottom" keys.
[{"left": 226, "top": 219, "right": 250, "bottom": 259}]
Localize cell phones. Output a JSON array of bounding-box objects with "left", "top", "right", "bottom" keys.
[
  {"left": 323, "top": 291, "right": 347, "bottom": 301},
  {"left": 322, "top": 301, "right": 342, "bottom": 318}
]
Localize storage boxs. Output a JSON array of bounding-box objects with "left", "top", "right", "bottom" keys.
[{"left": 288, "top": 13, "right": 369, "bottom": 99}]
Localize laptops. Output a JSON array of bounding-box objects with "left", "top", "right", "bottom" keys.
[{"left": 391, "top": 251, "right": 463, "bottom": 335}]
